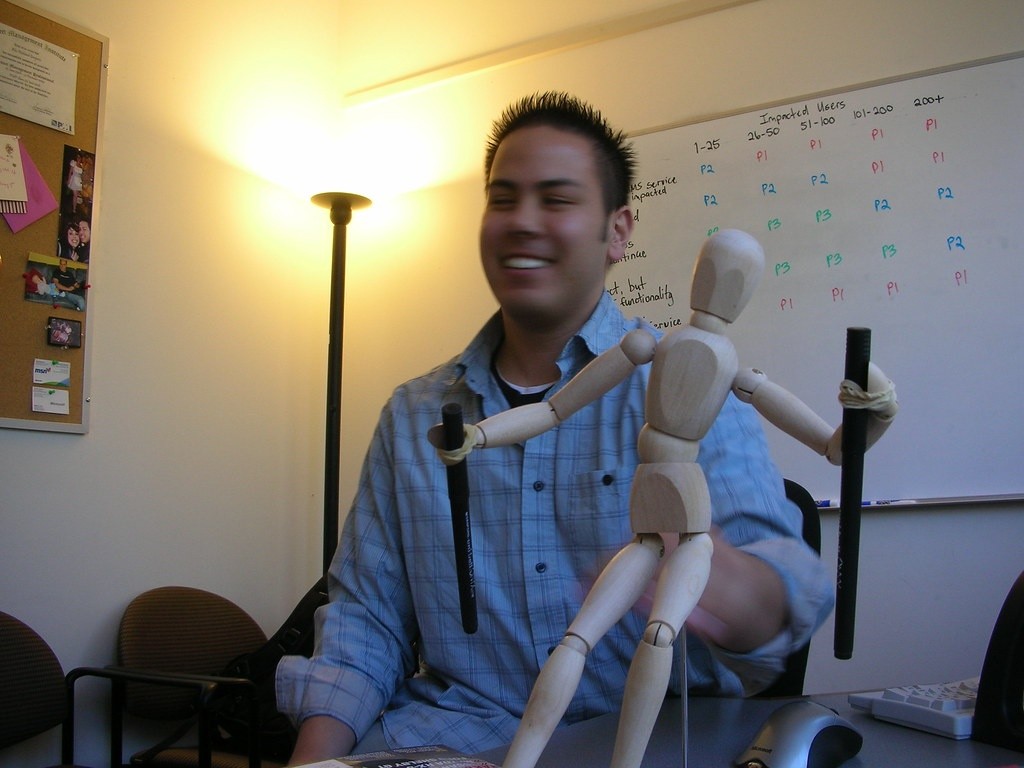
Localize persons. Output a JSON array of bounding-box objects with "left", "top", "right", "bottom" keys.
[
  {"left": 436, "top": 230, "right": 900, "bottom": 768},
  {"left": 282, "top": 87, "right": 834, "bottom": 766},
  {"left": 32, "top": 259, "right": 85, "bottom": 311},
  {"left": 63, "top": 152, "right": 95, "bottom": 261}
]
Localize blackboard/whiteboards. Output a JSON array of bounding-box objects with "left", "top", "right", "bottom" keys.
[{"left": 605, "top": 49, "right": 1024, "bottom": 510}]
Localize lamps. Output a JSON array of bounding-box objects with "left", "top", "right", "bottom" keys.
[{"left": 310, "top": 165, "right": 374, "bottom": 580}]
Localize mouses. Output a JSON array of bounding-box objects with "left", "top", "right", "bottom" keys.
[{"left": 735, "top": 700, "right": 864, "bottom": 768}]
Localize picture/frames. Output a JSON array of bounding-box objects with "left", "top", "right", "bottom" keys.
[{"left": 47, "top": 316, "right": 82, "bottom": 349}]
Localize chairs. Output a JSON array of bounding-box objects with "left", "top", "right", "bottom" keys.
[{"left": 0, "top": 585, "right": 269, "bottom": 768}]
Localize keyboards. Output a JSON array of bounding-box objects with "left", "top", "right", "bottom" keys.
[{"left": 849, "top": 676, "right": 981, "bottom": 740}]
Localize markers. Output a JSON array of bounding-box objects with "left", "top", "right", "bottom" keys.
[
  {"left": 815, "top": 499, "right": 842, "bottom": 508},
  {"left": 860, "top": 499, "right": 916, "bottom": 506}
]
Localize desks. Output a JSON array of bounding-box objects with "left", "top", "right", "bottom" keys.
[{"left": 468, "top": 689, "right": 1024, "bottom": 768}]
